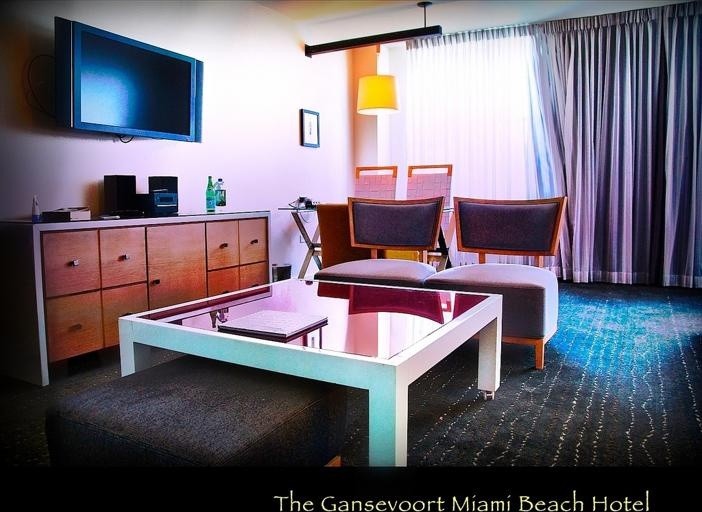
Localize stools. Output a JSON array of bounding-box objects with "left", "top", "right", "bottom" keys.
[{"left": 42, "top": 353, "right": 343, "bottom": 490}]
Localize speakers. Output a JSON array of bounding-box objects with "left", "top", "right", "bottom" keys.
[
  {"left": 103, "top": 171, "right": 137, "bottom": 215},
  {"left": 148, "top": 174, "right": 180, "bottom": 213}
]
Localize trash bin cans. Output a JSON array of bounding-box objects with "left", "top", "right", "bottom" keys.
[{"left": 272, "top": 263, "right": 292, "bottom": 285}]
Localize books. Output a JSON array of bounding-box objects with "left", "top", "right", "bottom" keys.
[
  {"left": 217, "top": 309, "right": 328, "bottom": 339},
  {"left": 42, "top": 206, "right": 92, "bottom": 221}
]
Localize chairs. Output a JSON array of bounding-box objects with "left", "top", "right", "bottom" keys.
[
  {"left": 313, "top": 194, "right": 446, "bottom": 287},
  {"left": 424, "top": 191, "right": 570, "bottom": 370},
  {"left": 406, "top": 164, "right": 454, "bottom": 241},
  {"left": 354, "top": 165, "right": 398, "bottom": 202},
  {"left": 315, "top": 203, "right": 388, "bottom": 271}
]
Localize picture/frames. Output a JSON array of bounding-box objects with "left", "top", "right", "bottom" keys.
[{"left": 299, "top": 108, "right": 321, "bottom": 149}]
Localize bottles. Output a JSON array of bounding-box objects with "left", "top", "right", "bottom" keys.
[
  {"left": 206, "top": 175, "right": 217, "bottom": 215},
  {"left": 32, "top": 194, "right": 40, "bottom": 222}
]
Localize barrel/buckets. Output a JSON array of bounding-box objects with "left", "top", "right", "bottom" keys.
[{"left": 212, "top": 178, "right": 227, "bottom": 212}]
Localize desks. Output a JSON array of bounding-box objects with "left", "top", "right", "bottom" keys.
[{"left": 278, "top": 204, "right": 454, "bottom": 280}]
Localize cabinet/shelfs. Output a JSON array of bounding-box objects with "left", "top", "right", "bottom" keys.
[
  {"left": 1, "top": 219, "right": 150, "bottom": 388},
  {"left": 146, "top": 222, "right": 207, "bottom": 306},
  {"left": 204, "top": 216, "right": 269, "bottom": 300}
]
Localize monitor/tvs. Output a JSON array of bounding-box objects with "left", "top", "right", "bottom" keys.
[{"left": 52, "top": 14, "right": 205, "bottom": 144}]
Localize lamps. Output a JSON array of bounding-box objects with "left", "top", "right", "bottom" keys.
[{"left": 356, "top": 74, "right": 402, "bottom": 116}]
